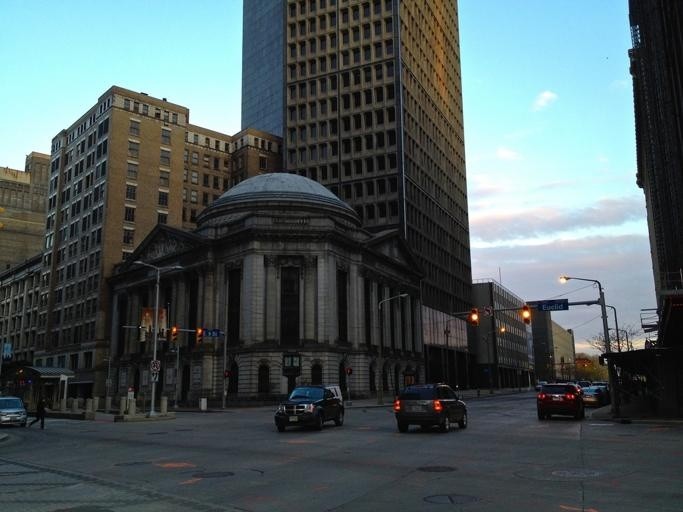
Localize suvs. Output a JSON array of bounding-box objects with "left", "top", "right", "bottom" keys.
[
  {"left": 275, "top": 384, "right": 347, "bottom": 434},
  {"left": 393, "top": 383, "right": 469, "bottom": 434},
  {"left": 535, "top": 383, "right": 587, "bottom": 422},
  {"left": 0, "top": 395, "right": 29, "bottom": 429}
]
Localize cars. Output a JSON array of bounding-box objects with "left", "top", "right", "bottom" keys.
[{"left": 535, "top": 380, "right": 613, "bottom": 410}]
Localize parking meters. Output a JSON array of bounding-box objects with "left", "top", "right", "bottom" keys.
[{"left": 345, "top": 368, "right": 352, "bottom": 406}]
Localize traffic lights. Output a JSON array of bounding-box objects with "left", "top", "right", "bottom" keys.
[
  {"left": 195, "top": 328, "right": 203, "bottom": 345},
  {"left": 598, "top": 356, "right": 606, "bottom": 367},
  {"left": 224, "top": 369, "right": 232, "bottom": 378},
  {"left": 471, "top": 308, "right": 480, "bottom": 328},
  {"left": 170, "top": 326, "right": 178, "bottom": 344},
  {"left": 522, "top": 305, "right": 531, "bottom": 326}
]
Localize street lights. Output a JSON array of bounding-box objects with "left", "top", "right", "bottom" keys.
[
  {"left": 101, "top": 358, "right": 110, "bottom": 395},
  {"left": 377, "top": 293, "right": 410, "bottom": 408},
  {"left": 585, "top": 300, "right": 622, "bottom": 353},
  {"left": 531, "top": 343, "right": 548, "bottom": 389},
  {"left": 443, "top": 315, "right": 470, "bottom": 386},
  {"left": 486, "top": 328, "right": 507, "bottom": 396},
  {"left": 606, "top": 327, "right": 631, "bottom": 352},
  {"left": 133, "top": 259, "right": 185, "bottom": 419},
  {"left": 558, "top": 275, "right": 619, "bottom": 415}
]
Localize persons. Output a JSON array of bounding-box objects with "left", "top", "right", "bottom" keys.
[{"left": 28, "top": 397, "right": 49, "bottom": 430}]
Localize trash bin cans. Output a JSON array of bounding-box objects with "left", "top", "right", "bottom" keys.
[{"left": 198, "top": 395, "right": 208, "bottom": 411}]
